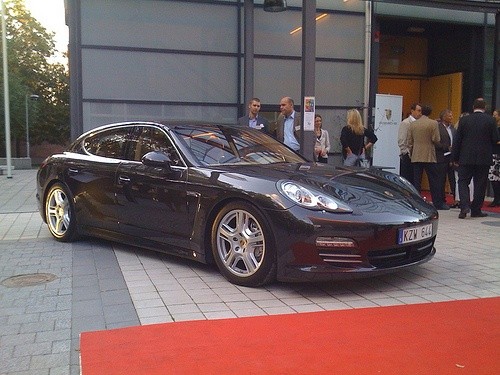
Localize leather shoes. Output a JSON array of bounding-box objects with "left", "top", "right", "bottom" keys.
[
  {"left": 459, "top": 208, "right": 469, "bottom": 218},
  {"left": 487, "top": 202, "right": 500, "bottom": 207},
  {"left": 435, "top": 203, "right": 450, "bottom": 209},
  {"left": 471, "top": 212, "right": 487, "bottom": 217}
]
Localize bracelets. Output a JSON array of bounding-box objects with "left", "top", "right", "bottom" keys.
[{"left": 363, "top": 144, "right": 367, "bottom": 151}]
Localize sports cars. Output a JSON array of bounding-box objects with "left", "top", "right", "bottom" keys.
[{"left": 37, "top": 119, "right": 438, "bottom": 286}]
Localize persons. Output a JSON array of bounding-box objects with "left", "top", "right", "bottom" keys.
[
  {"left": 274, "top": 97, "right": 301, "bottom": 157},
  {"left": 486, "top": 106, "right": 500, "bottom": 207},
  {"left": 407, "top": 104, "right": 449, "bottom": 210},
  {"left": 436, "top": 109, "right": 459, "bottom": 208},
  {"left": 313, "top": 113, "right": 330, "bottom": 164},
  {"left": 397, "top": 103, "right": 422, "bottom": 184},
  {"left": 235, "top": 98, "right": 270, "bottom": 135},
  {"left": 451, "top": 97, "right": 499, "bottom": 218}
]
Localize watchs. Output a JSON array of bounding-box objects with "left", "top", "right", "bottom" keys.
[{"left": 340, "top": 108, "right": 378, "bottom": 169}]
